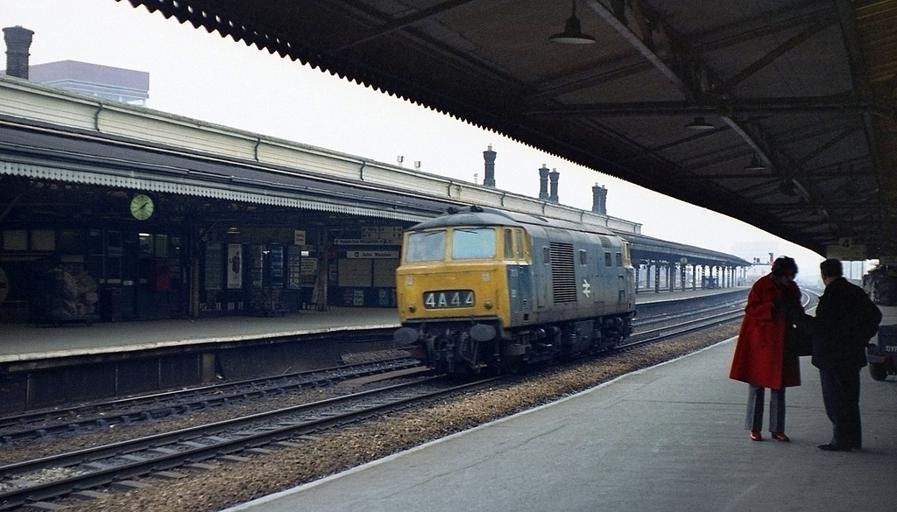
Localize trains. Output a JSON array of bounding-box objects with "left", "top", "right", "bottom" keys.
[{"left": 396, "top": 204, "right": 638, "bottom": 377}]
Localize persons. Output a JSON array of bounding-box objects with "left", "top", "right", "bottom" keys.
[
  {"left": 232, "top": 249, "right": 240, "bottom": 284},
  {"left": 728, "top": 256, "right": 883, "bottom": 452}
]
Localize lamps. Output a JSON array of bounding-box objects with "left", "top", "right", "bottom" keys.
[
  {"left": 742, "top": 150, "right": 765, "bottom": 170},
  {"left": 546, "top": 1, "right": 597, "bottom": 48},
  {"left": 683, "top": 111, "right": 716, "bottom": 132}
]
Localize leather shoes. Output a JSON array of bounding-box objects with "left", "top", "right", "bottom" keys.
[
  {"left": 817, "top": 442, "right": 861, "bottom": 451},
  {"left": 772, "top": 432, "right": 789, "bottom": 442},
  {"left": 750, "top": 432, "right": 761, "bottom": 441}
]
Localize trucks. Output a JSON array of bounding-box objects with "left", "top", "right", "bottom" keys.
[{"left": 866, "top": 273, "right": 897, "bottom": 381}]
